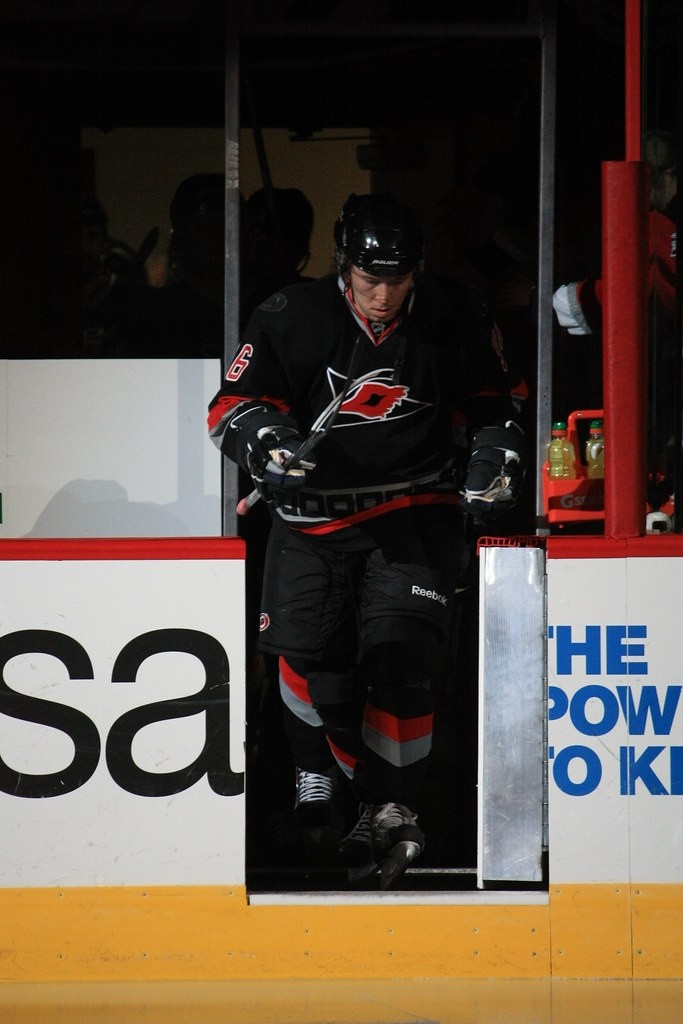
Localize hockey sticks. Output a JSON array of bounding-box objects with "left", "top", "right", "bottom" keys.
[{"left": 233, "top": 328, "right": 370, "bottom": 513}]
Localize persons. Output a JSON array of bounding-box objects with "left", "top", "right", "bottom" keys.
[
  {"left": 2, "top": 65, "right": 682, "bottom": 541},
  {"left": 208, "top": 193, "right": 531, "bottom": 893}
]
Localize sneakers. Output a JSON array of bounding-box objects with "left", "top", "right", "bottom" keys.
[
  {"left": 290, "top": 761, "right": 340, "bottom": 857},
  {"left": 336, "top": 798, "right": 425, "bottom": 890}
]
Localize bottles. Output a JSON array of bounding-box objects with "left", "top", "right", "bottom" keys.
[
  {"left": 549, "top": 421, "right": 576, "bottom": 481},
  {"left": 586, "top": 419, "right": 604, "bottom": 479}
]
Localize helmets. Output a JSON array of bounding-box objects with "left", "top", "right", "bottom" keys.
[{"left": 332, "top": 191, "right": 426, "bottom": 279}]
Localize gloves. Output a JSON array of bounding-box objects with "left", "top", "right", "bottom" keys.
[
  {"left": 461, "top": 418, "right": 525, "bottom": 518},
  {"left": 234, "top": 412, "right": 319, "bottom": 495}
]
